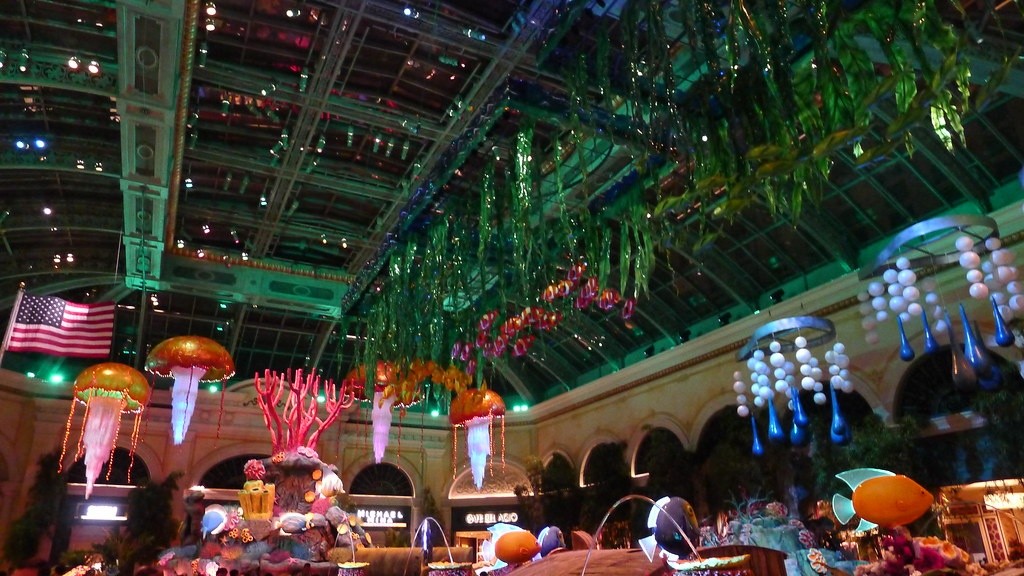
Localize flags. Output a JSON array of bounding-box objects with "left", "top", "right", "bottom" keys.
[{"left": 5, "top": 292, "right": 115, "bottom": 359}]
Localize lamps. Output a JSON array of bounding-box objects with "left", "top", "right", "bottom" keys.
[
  {"left": 644, "top": 345, "right": 655, "bottom": 358},
  {"left": 286, "top": 3, "right": 304, "bottom": 17},
  {"left": 680, "top": 330, "right": 691, "bottom": 343},
  {"left": 719, "top": 312, "right": 732, "bottom": 327},
  {"left": 403, "top": 4, "right": 423, "bottom": 20},
  {"left": 769, "top": 290, "right": 784, "bottom": 305},
  {"left": 187, "top": 109, "right": 200, "bottom": 128},
  {"left": 69, "top": 56, "right": 101, "bottom": 75},
  {"left": 261, "top": 81, "right": 279, "bottom": 96},
  {"left": 220, "top": 96, "right": 231, "bottom": 116},
  {"left": 205, "top": 0, "right": 216, "bottom": 15},
  {"left": 205, "top": 17, "right": 216, "bottom": 31}
]
